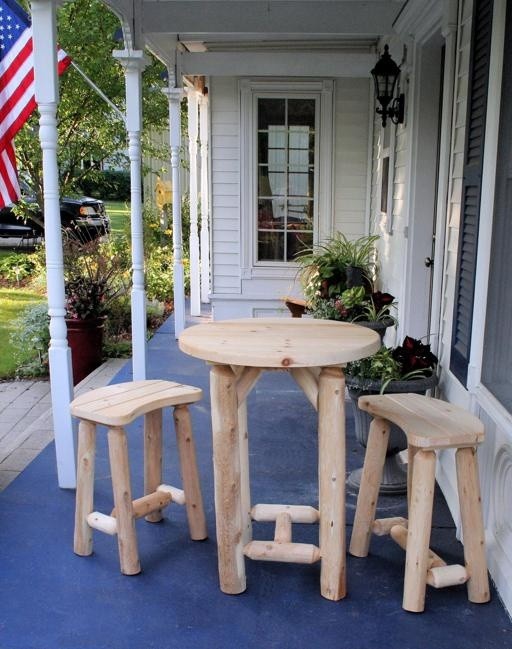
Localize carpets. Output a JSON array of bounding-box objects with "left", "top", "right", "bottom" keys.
[{"left": 0, "top": 297, "right": 512, "bottom": 649}]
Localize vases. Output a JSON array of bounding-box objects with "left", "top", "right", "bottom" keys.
[
  {"left": 343, "top": 374, "right": 440, "bottom": 455},
  {"left": 343, "top": 337, "right": 447, "bottom": 398},
  {"left": 65, "top": 317, "right": 103, "bottom": 389},
  {"left": 358, "top": 316, "right": 394, "bottom": 341}
]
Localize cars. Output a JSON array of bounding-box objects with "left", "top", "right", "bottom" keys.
[{"left": 0, "top": 176, "right": 113, "bottom": 254}]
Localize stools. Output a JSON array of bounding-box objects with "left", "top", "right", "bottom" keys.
[
  {"left": 357, "top": 391, "right": 493, "bottom": 613},
  {"left": 67, "top": 378, "right": 206, "bottom": 578}
]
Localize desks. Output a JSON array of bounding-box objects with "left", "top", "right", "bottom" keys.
[{"left": 178, "top": 318, "right": 384, "bottom": 602}]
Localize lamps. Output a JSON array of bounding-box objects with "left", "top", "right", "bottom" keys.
[{"left": 369, "top": 43, "right": 404, "bottom": 127}]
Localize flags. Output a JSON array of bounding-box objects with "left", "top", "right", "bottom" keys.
[{"left": 0, "top": 0, "right": 72, "bottom": 211}]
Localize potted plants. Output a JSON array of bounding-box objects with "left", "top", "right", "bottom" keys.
[{"left": 292, "top": 229, "right": 375, "bottom": 293}]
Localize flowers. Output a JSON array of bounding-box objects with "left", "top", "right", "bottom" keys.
[
  {"left": 307, "top": 286, "right": 402, "bottom": 324},
  {"left": 55, "top": 216, "right": 133, "bottom": 318}
]
